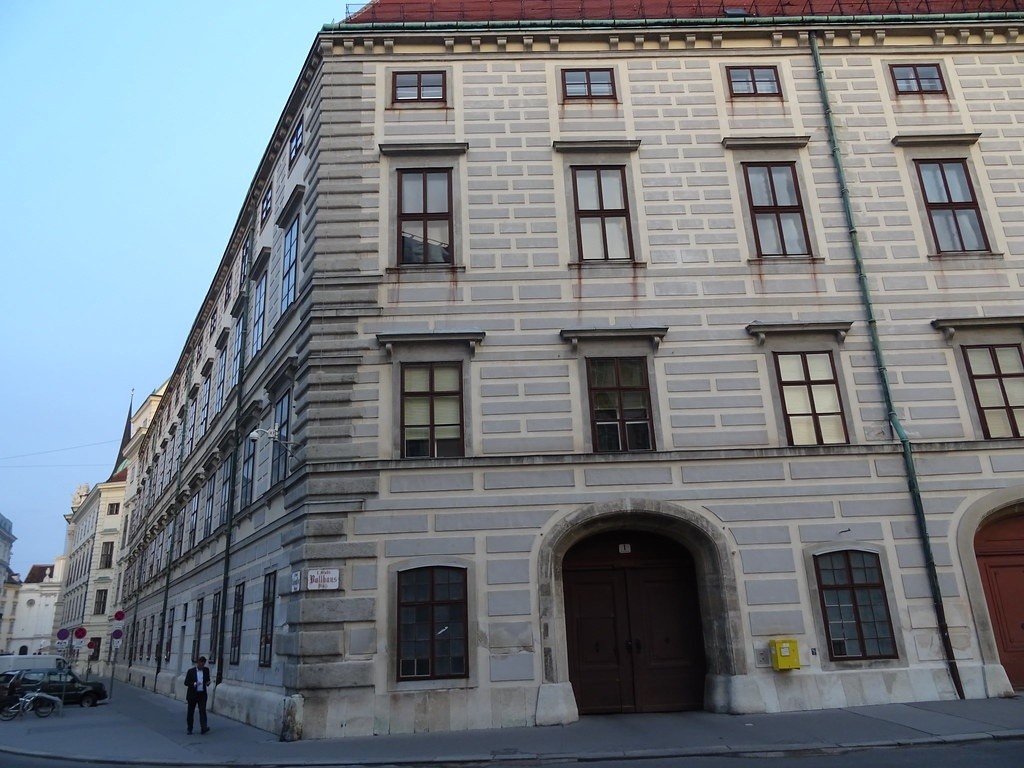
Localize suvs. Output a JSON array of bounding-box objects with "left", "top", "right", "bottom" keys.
[{"left": 0, "top": 666, "right": 109, "bottom": 707}]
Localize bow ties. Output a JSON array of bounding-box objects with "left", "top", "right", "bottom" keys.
[{"left": 198, "top": 667, "right": 204, "bottom": 671}]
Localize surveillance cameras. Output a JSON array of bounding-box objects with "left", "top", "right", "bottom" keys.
[{"left": 250, "top": 432, "right": 260, "bottom": 442}]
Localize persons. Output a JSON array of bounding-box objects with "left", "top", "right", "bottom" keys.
[{"left": 184, "top": 656, "right": 211, "bottom": 735}]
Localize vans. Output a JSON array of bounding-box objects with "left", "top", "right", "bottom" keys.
[{"left": 0, "top": 654, "right": 74, "bottom": 682}]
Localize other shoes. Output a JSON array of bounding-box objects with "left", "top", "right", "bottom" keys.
[
  {"left": 201, "top": 727, "right": 210, "bottom": 734},
  {"left": 186, "top": 729, "right": 192, "bottom": 735}
]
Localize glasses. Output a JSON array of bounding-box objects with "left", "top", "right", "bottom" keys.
[{"left": 201, "top": 660, "right": 206, "bottom": 662}]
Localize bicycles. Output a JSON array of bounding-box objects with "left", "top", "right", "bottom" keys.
[{"left": 0, "top": 682, "right": 58, "bottom": 722}]
